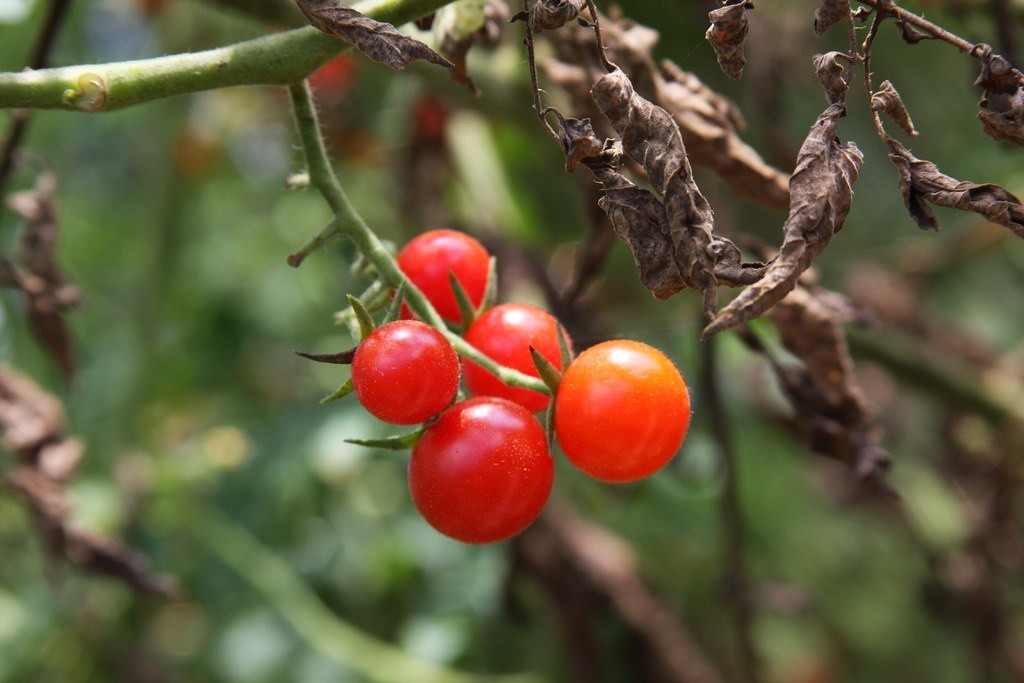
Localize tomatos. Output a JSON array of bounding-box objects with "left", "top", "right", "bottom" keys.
[{"left": 351, "top": 226, "right": 694, "bottom": 545}]
